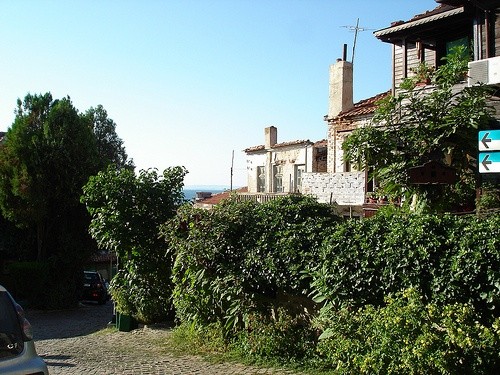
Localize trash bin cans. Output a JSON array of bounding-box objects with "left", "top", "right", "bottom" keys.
[
  {"left": 119, "top": 311, "right": 131, "bottom": 332},
  {"left": 116, "top": 311, "right": 121, "bottom": 329}
]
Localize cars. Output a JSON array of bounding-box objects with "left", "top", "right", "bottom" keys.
[
  {"left": 81, "top": 271, "right": 107, "bottom": 306},
  {"left": 0, "top": 286, "right": 50, "bottom": 375}
]
